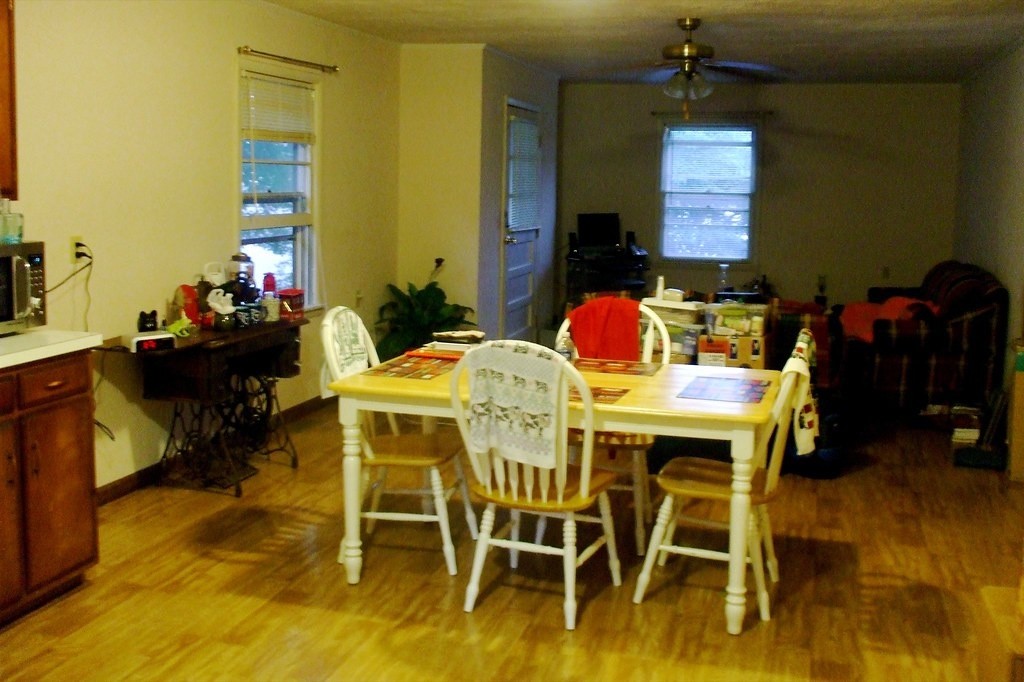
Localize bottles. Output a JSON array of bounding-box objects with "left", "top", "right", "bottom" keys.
[
  {"left": 229, "top": 252, "right": 253, "bottom": 282},
  {"left": 0, "top": 198, "right": 23, "bottom": 244}
]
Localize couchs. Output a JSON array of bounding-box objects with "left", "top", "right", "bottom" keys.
[{"left": 838, "top": 259, "right": 1009, "bottom": 433}]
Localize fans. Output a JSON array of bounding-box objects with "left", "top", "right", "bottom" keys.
[{"left": 569, "top": 16, "right": 801, "bottom": 85}]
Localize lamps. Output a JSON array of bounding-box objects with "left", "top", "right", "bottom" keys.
[{"left": 661, "top": 16, "right": 713, "bottom": 102}]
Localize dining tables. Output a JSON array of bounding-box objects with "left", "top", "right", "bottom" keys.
[{"left": 327, "top": 339, "right": 783, "bottom": 635}]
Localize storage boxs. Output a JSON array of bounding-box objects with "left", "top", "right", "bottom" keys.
[{"left": 637, "top": 295, "right": 774, "bottom": 371}]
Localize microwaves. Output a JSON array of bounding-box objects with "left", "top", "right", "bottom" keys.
[{"left": 0, "top": 241, "right": 48, "bottom": 337}]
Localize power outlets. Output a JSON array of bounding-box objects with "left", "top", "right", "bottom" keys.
[{"left": 71, "top": 235, "right": 84, "bottom": 264}]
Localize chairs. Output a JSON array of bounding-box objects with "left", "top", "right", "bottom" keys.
[
  {"left": 534, "top": 297, "right": 671, "bottom": 556},
  {"left": 633, "top": 328, "right": 813, "bottom": 622},
  {"left": 320, "top": 306, "right": 479, "bottom": 576},
  {"left": 449, "top": 339, "right": 622, "bottom": 630}
]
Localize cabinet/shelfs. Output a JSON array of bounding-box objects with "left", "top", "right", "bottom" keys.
[
  {"left": 565, "top": 246, "right": 648, "bottom": 312},
  {"left": 0, "top": 0, "right": 17, "bottom": 200},
  {"left": 0, "top": 347, "right": 99, "bottom": 629}
]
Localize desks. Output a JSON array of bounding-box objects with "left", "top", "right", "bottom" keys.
[{"left": 139, "top": 318, "right": 311, "bottom": 498}]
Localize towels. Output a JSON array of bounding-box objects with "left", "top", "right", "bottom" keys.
[
  {"left": 465, "top": 340, "right": 566, "bottom": 469},
  {"left": 772, "top": 329, "right": 820, "bottom": 457},
  {"left": 319, "top": 304, "right": 368, "bottom": 399},
  {"left": 567, "top": 296, "right": 639, "bottom": 360}
]
